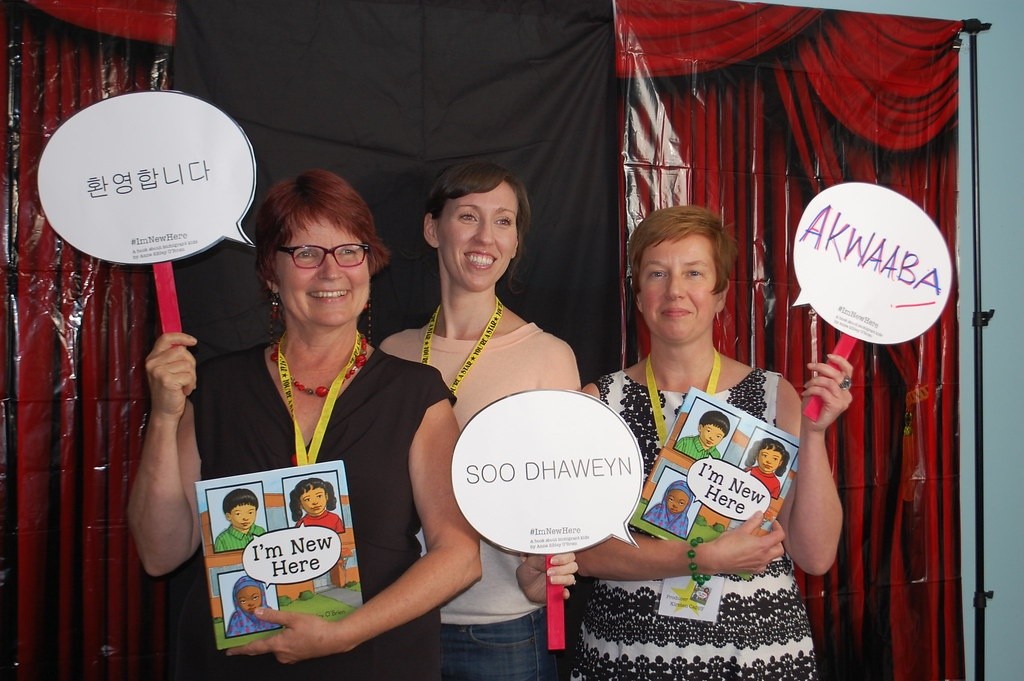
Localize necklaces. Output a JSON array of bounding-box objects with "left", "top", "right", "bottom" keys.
[{"left": 271, "top": 333, "right": 367, "bottom": 396}]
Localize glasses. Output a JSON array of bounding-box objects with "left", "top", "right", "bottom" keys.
[{"left": 277, "top": 243, "right": 370, "bottom": 268}]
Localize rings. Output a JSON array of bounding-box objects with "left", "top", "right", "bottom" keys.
[{"left": 840, "top": 374, "right": 852, "bottom": 390}]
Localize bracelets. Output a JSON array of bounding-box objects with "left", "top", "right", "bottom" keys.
[{"left": 687, "top": 536, "right": 712, "bottom": 585}]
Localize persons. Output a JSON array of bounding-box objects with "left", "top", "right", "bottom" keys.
[
  {"left": 380, "top": 161, "right": 581, "bottom": 681},
  {"left": 126, "top": 170, "right": 482, "bottom": 681},
  {"left": 565, "top": 205, "right": 852, "bottom": 681}
]
